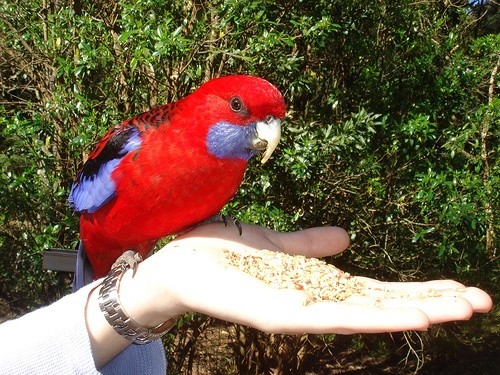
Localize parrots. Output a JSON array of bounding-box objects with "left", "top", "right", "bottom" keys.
[{"left": 66, "top": 75, "right": 287, "bottom": 293}]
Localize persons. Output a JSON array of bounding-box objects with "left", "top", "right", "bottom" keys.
[{"left": 0, "top": 222, "right": 493, "bottom": 375}]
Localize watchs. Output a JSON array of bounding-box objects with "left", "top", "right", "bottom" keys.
[{"left": 98, "top": 267, "right": 177, "bottom": 344}]
